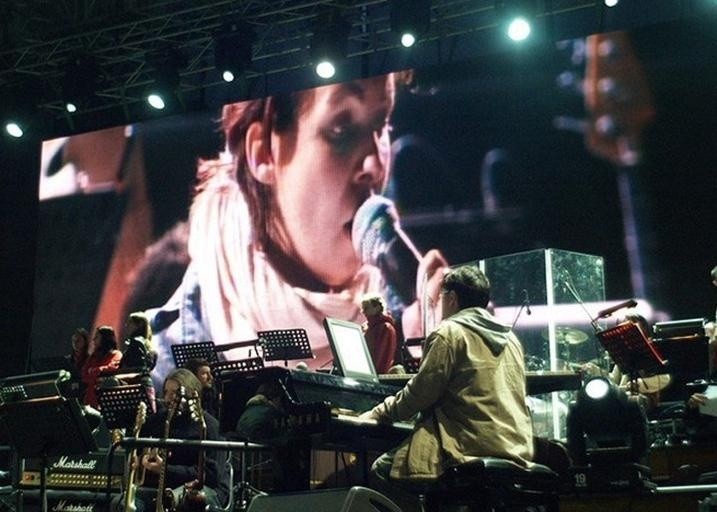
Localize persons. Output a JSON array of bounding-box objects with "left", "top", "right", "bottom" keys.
[
  {"left": 183, "top": 357, "right": 213, "bottom": 390},
  {"left": 389, "top": 363, "right": 407, "bottom": 375},
  {"left": 117, "top": 315, "right": 159, "bottom": 417},
  {"left": 358, "top": 291, "right": 397, "bottom": 376},
  {"left": 130, "top": 369, "right": 227, "bottom": 511},
  {"left": 309, "top": 264, "right": 534, "bottom": 506},
  {"left": 62, "top": 325, "right": 89, "bottom": 371},
  {"left": 686, "top": 384, "right": 717, "bottom": 428},
  {"left": 297, "top": 361, "right": 308, "bottom": 369},
  {"left": 125, "top": 68, "right": 455, "bottom": 376},
  {"left": 581, "top": 312, "right": 662, "bottom": 413},
  {"left": 85, "top": 327, "right": 124, "bottom": 413}
]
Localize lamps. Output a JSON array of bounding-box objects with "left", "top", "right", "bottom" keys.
[
  {"left": 576, "top": 374, "right": 641, "bottom": 449},
  {"left": 1, "top": 0, "right": 538, "bottom": 141}
]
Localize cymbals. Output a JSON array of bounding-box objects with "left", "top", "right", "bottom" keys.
[
  {"left": 542, "top": 326, "right": 587, "bottom": 345},
  {"left": 629, "top": 371, "right": 669, "bottom": 392}
]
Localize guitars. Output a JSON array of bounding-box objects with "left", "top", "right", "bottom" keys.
[
  {"left": 179, "top": 391, "right": 222, "bottom": 512},
  {"left": 555, "top": 32, "right": 674, "bottom": 302},
  {"left": 109, "top": 401, "right": 151, "bottom": 512},
  {"left": 142, "top": 387, "right": 180, "bottom": 512}
]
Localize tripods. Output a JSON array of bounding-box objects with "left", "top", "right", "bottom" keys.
[{"left": 230, "top": 450, "right": 270, "bottom": 511}]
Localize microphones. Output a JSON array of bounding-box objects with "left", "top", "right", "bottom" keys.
[
  {"left": 350, "top": 194, "right": 424, "bottom": 307},
  {"left": 522, "top": 288, "right": 532, "bottom": 317}
]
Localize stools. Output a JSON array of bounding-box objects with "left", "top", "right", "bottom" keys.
[{"left": 379, "top": 456, "right": 560, "bottom": 512}]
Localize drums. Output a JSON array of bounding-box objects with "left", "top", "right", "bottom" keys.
[
  {"left": 524, "top": 356, "right": 546, "bottom": 371},
  {"left": 539, "top": 357, "right": 573, "bottom": 401}
]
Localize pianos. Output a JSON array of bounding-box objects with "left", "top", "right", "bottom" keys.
[{"left": 222, "top": 369, "right": 420, "bottom": 498}]
